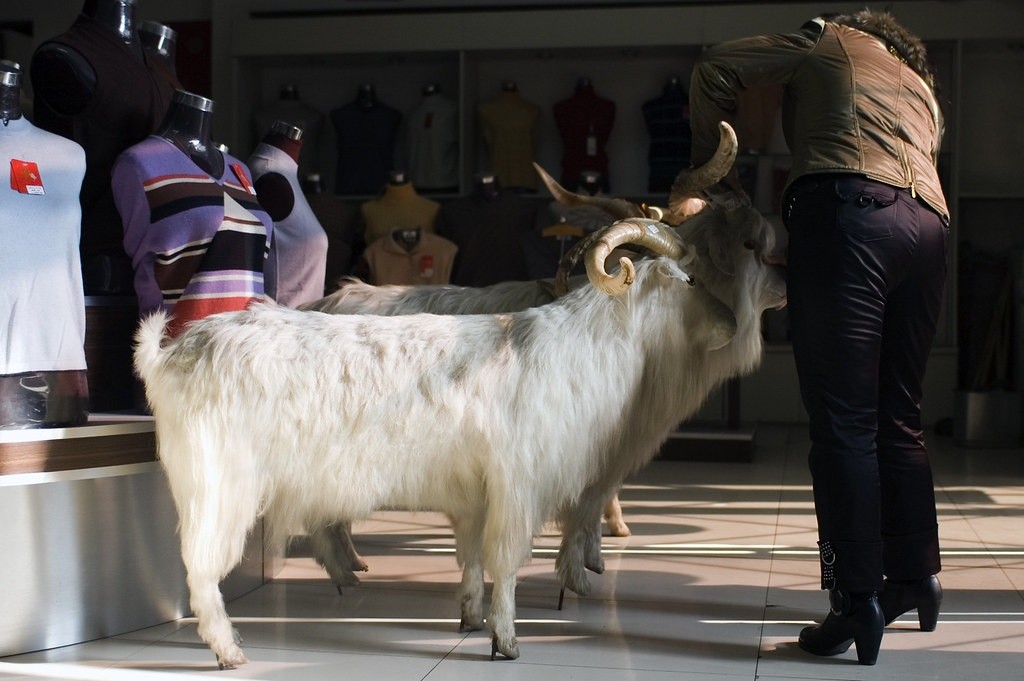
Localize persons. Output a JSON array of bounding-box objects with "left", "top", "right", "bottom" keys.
[
  {"left": 0, "top": 1, "right": 329, "bottom": 430},
  {"left": 393, "top": 78, "right": 457, "bottom": 187},
  {"left": 249, "top": 83, "right": 338, "bottom": 194},
  {"left": 642, "top": 72, "right": 693, "bottom": 194},
  {"left": 687, "top": 7, "right": 951, "bottom": 666},
  {"left": 300, "top": 171, "right": 535, "bottom": 294},
  {"left": 553, "top": 77, "right": 617, "bottom": 196},
  {"left": 330, "top": 81, "right": 402, "bottom": 195},
  {"left": 480, "top": 81, "right": 538, "bottom": 195}
]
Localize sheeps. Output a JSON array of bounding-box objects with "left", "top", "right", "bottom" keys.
[
  {"left": 292, "top": 119, "right": 786, "bottom": 587},
  {"left": 133, "top": 220, "right": 738, "bottom": 670}
]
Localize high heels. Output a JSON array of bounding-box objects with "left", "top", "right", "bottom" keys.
[
  {"left": 799, "top": 588, "right": 886, "bottom": 665},
  {"left": 881, "top": 571, "right": 942, "bottom": 632}
]
